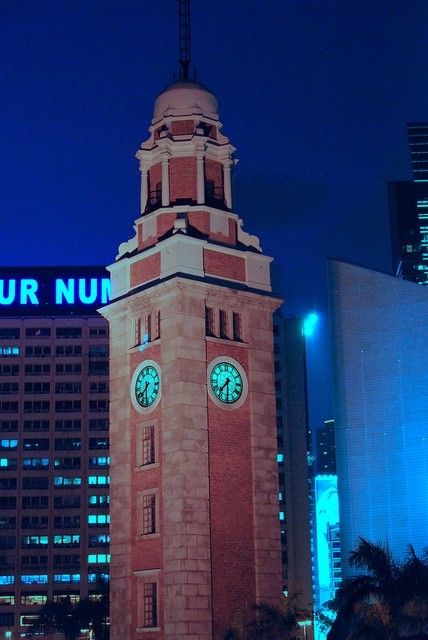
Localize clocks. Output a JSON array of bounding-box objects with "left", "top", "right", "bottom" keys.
[
  {"left": 207, "top": 356, "right": 249, "bottom": 410},
  {"left": 129, "top": 358, "right": 163, "bottom": 415}
]
